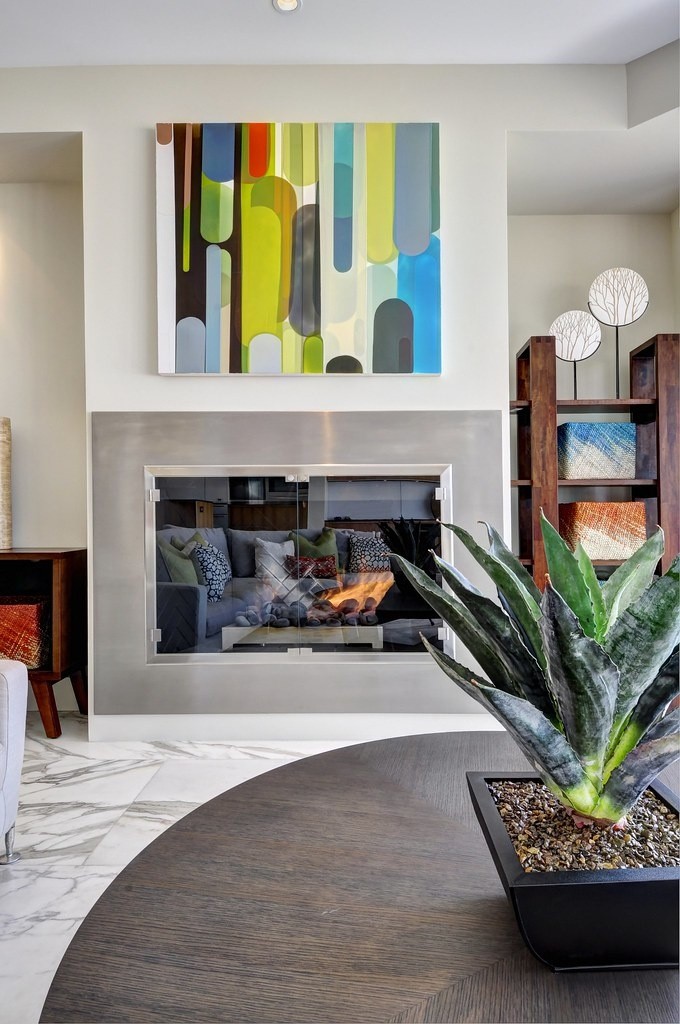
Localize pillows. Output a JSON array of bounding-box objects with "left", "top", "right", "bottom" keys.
[
  {"left": 253, "top": 537, "right": 295, "bottom": 578},
  {"left": 287, "top": 529, "right": 340, "bottom": 570},
  {"left": 156, "top": 531, "right": 232, "bottom": 603},
  {"left": 349, "top": 535, "right": 392, "bottom": 573},
  {"left": 322, "top": 527, "right": 375, "bottom": 568},
  {"left": 286, "top": 554, "right": 338, "bottom": 579}
]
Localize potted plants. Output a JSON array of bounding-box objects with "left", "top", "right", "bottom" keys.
[{"left": 386, "top": 509, "right": 680, "bottom": 969}]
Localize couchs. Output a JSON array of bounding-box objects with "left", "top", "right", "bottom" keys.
[
  {"left": 156, "top": 526, "right": 444, "bottom": 654},
  {"left": 0, "top": 659, "right": 28, "bottom": 865}
]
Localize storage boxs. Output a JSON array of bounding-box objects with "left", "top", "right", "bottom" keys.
[
  {"left": 0, "top": 593, "right": 51, "bottom": 672},
  {"left": 556, "top": 421, "right": 637, "bottom": 480},
  {"left": 558, "top": 500, "right": 647, "bottom": 561}
]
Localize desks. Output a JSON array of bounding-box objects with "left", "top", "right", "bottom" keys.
[
  {"left": 221, "top": 623, "right": 383, "bottom": 652},
  {"left": 37, "top": 730, "right": 680, "bottom": 1024},
  {"left": 0, "top": 547, "right": 88, "bottom": 739}
]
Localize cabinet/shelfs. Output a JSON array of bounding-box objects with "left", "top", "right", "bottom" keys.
[{"left": 510, "top": 334, "right": 680, "bottom": 595}]
